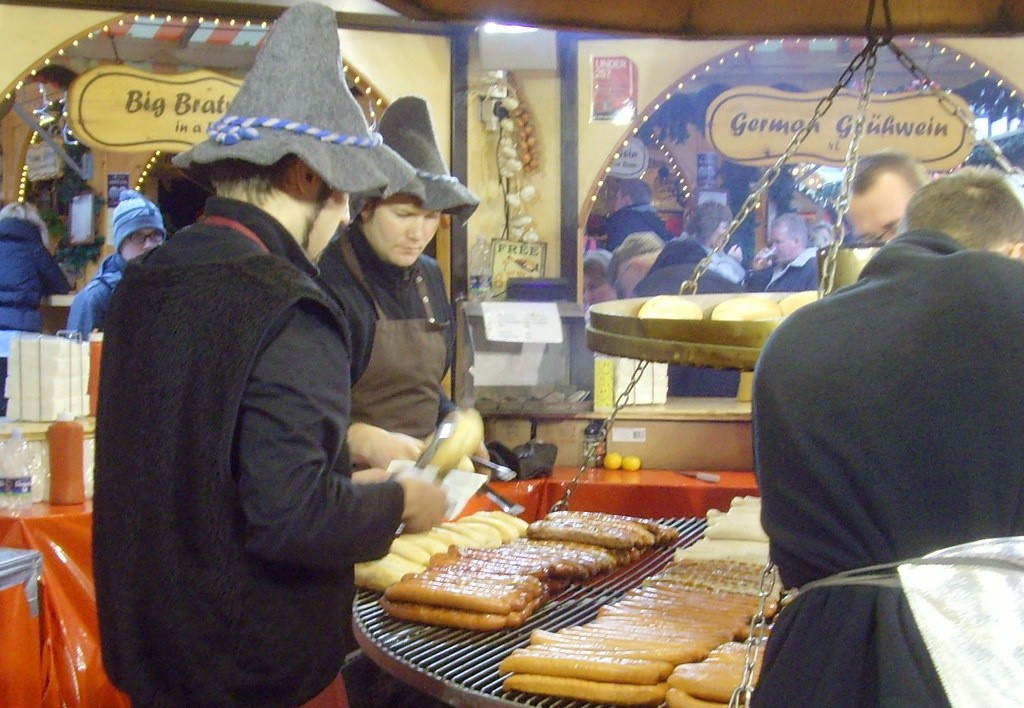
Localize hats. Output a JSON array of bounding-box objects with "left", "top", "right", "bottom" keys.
[
  {"left": 112, "top": 189, "right": 167, "bottom": 255},
  {"left": 374, "top": 96, "right": 480, "bottom": 226},
  {"left": 171, "top": 2, "right": 418, "bottom": 201}
]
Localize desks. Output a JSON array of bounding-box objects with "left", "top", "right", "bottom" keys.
[{"left": 477, "top": 395, "right": 754, "bottom": 469}]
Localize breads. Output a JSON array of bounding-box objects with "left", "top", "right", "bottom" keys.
[
  {"left": 672, "top": 493, "right": 771, "bottom": 566},
  {"left": 353, "top": 404, "right": 533, "bottom": 591},
  {"left": 636, "top": 290, "right": 825, "bottom": 321}
]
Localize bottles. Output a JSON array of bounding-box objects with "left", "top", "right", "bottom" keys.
[
  {"left": 0, "top": 426, "right": 33, "bottom": 516},
  {"left": 470, "top": 235, "right": 494, "bottom": 303},
  {"left": 86, "top": 329, "right": 105, "bottom": 416},
  {"left": 46, "top": 410, "right": 84, "bottom": 505}
]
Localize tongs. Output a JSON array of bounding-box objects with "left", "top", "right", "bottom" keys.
[
  {"left": 471, "top": 456, "right": 525, "bottom": 515},
  {"left": 395, "top": 418, "right": 460, "bottom": 540}
]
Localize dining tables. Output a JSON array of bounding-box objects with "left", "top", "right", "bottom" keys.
[{"left": 352, "top": 516, "right": 824, "bottom": 708}]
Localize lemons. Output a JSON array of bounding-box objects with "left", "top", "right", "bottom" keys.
[{"left": 603, "top": 452, "right": 641, "bottom": 470}]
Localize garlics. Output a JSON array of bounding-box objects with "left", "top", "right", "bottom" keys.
[{"left": 497, "top": 118, "right": 539, "bottom": 244}]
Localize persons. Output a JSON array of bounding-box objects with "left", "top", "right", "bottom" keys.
[
  {"left": 91, "top": 2, "right": 450, "bottom": 708},
  {"left": 581, "top": 174, "right": 834, "bottom": 398},
  {"left": 318, "top": 97, "right": 493, "bottom": 490},
  {"left": 842, "top": 148, "right": 928, "bottom": 243},
  {"left": 65, "top": 190, "right": 165, "bottom": 341},
  {"left": 750, "top": 168, "right": 1024, "bottom": 708},
  {"left": 0, "top": 200, "right": 70, "bottom": 416}
]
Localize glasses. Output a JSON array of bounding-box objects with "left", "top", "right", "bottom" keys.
[{"left": 125, "top": 229, "right": 165, "bottom": 245}]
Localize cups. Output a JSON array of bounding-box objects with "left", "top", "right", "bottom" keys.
[{"left": 753, "top": 246, "right": 783, "bottom": 270}]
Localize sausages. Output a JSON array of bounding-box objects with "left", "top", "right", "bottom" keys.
[
  {"left": 380, "top": 508, "right": 683, "bottom": 630},
  {"left": 500, "top": 556, "right": 788, "bottom": 708}
]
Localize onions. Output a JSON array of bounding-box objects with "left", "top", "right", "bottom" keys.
[{"left": 513, "top": 106, "right": 539, "bottom": 171}]
